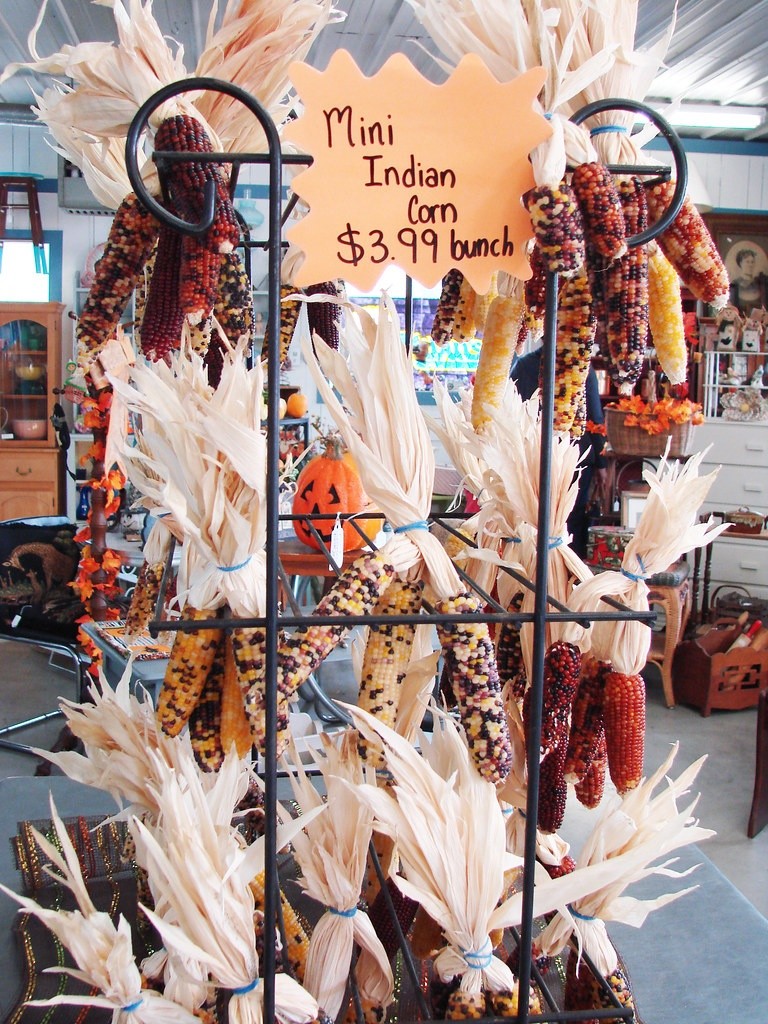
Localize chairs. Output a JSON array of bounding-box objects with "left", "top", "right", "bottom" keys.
[{"left": 1, "top": 516, "right": 98, "bottom": 762}]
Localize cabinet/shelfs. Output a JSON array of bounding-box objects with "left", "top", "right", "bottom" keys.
[
  {"left": 65, "top": 431, "right": 97, "bottom": 530},
  {"left": 0, "top": 301, "right": 73, "bottom": 520},
  {"left": 687, "top": 414, "right": 768, "bottom": 607}
]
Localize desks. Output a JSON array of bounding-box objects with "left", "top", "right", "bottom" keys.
[
  {"left": 584, "top": 575, "right": 693, "bottom": 710},
  {"left": 83, "top": 620, "right": 176, "bottom": 713}
]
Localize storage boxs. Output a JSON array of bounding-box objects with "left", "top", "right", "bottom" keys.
[
  {"left": 586, "top": 525, "right": 639, "bottom": 571},
  {"left": 677, "top": 640, "right": 768, "bottom": 718}
]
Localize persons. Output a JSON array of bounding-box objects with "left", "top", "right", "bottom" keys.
[
  {"left": 727, "top": 248, "right": 768, "bottom": 322},
  {"left": 508, "top": 333, "right": 608, "bottom": 561}
]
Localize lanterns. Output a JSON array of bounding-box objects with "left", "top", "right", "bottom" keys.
[{"left": 292, "top": 433, "right": 387, "bottom": 553}]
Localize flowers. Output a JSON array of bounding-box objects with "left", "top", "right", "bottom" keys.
[{"left": 601, "top": 392, "right": 705, "bottom": 433}]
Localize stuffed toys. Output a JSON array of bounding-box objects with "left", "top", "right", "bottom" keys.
[{"left": 715, "top": 302, "right": 768, "bottom": 352}]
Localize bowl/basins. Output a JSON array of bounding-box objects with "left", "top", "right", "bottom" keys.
[
  {"left": 11, "top": 419, "right": 47, "bottom": 439},
  {"left": 15, "top": 366, "right": 43, "bottom": 380}
]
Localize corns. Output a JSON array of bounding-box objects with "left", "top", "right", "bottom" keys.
[{"left": 70, "top": 115, "right": 731, "bottom": 1024}]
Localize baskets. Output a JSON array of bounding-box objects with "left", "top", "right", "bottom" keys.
[{"left": 603, "top": 404, "right": 694, "bottom": 457}]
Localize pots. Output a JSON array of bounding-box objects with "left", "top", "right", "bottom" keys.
[{"left": 725, "top": 506, "right": 766, "bottom": 535}]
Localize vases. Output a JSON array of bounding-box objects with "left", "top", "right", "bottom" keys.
[{"left": 77, "top": 486, "right": 91, "bottom": 520}]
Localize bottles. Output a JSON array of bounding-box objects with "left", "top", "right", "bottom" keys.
[{"left": 77, "top": 488, "right": 90, "bottom": 519}]
